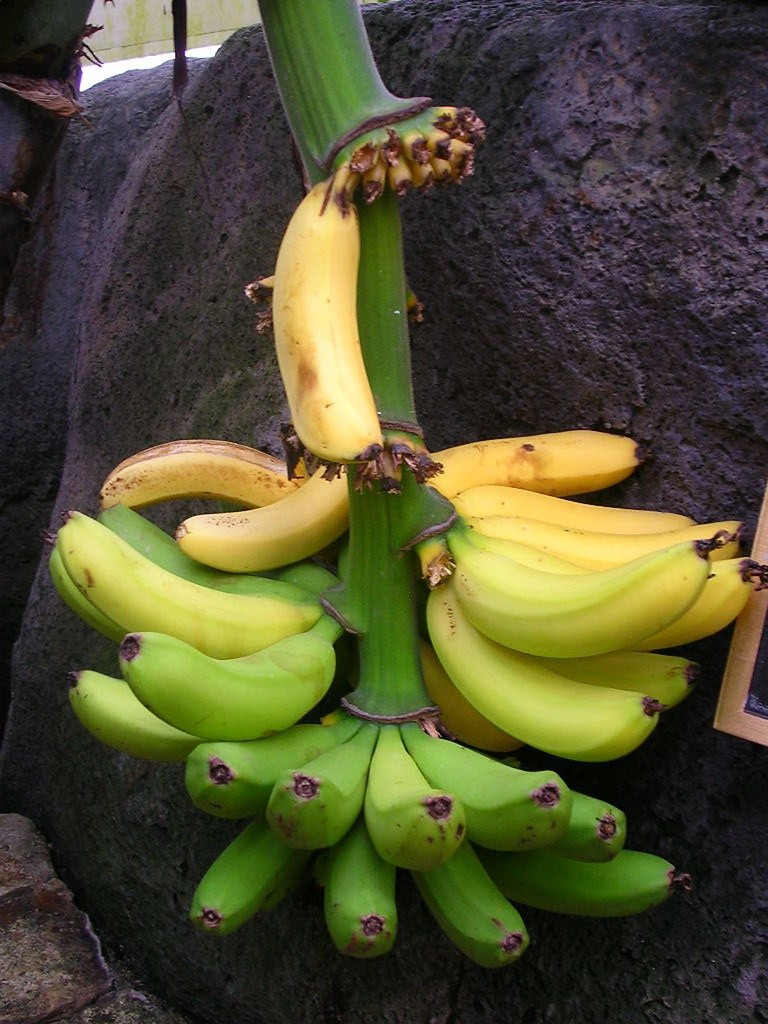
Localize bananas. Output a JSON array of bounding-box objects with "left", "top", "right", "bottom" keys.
[{"left": 51, "top": 178, "right": 767, "bottom": 965}]
